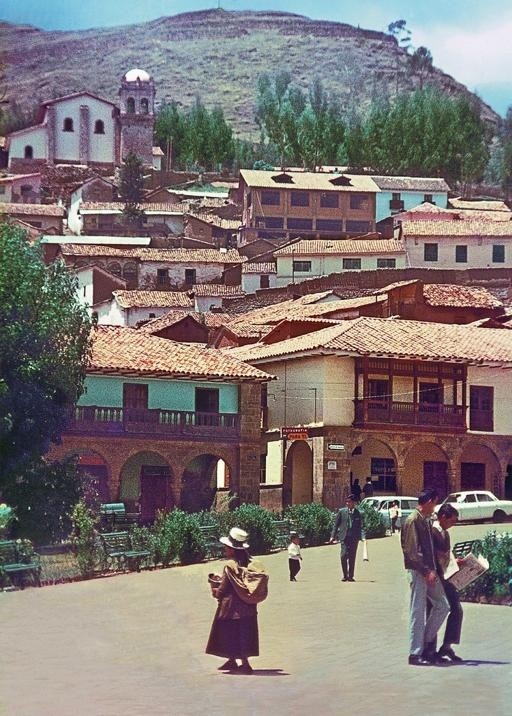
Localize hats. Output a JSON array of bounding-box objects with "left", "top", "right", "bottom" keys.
[{"left": 219, "top": 526, "right": 252, "bottom": 552}]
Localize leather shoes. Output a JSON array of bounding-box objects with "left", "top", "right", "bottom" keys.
[
  {"left": 408, "top": 653, "right": 434, "bottom": 667},
  {"left": 422, "top": 649, "right": 448, "bottom": 664},
  {"left": 438, "top": 646, "right": 462, "bottom": 662}
]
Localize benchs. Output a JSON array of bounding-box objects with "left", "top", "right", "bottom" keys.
[
  {"left": 270, "top": 519, "right": 291, "bottom": 550},
  {"left": 453, "top": 538, "right": 482, "bottom": 561},
  {"left": 98, "top": 529, "right": 151, "bottom": 572},
  {"left": 199, "top": 525, "right": 224, "bottom": 560},
  {"left": 1, "top": 539, "right": 42, "bottom": 590},
  {"left": 98, "top": 502, "right": 140, "bottom": 528}
]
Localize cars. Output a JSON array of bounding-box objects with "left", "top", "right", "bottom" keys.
[
  {"left": 433, "top": 490, "right": 512, "bottom": 524},
  {"left": 338, "top": 495, "right": 437, "bottom": 531}
]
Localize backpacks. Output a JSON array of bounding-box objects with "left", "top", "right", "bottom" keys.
[{"left": 224, "top": 557, "right": 270, "bottom": 605}]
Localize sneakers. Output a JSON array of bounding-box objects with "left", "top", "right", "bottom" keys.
[
  {"left": 218, "top": 661, "right": 238, "bottom": 670},
  {"left": 236, "top": 663, "right": 254, "bottom": 675}
]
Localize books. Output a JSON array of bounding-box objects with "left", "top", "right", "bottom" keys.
[{"left": 446, "top": 553, "right": 491, "bottom": 590}]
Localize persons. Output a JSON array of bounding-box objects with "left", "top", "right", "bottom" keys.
[
  {"left": 351, "top": 479, "right": 361, "bottom": 503},
  {"left": 423, "top": 504, "right": 466, "bottom": 664},
  {"left": 392, "top": 500, "right": 401, "bottom": 534},
  {"left": 362, "top": 477, "right": 373, "bottom": 498},
  {"left": 328, "top": 495, "right": 363, "bottom": 582},
  {"left": 287, "top": 534, "right": 302, "bottom": 581},
  {"left": 400, "top": 489, "right": 451, "bottom": 666},
  {"left": 206, "top": 525, "right": 263, "bottom": 673}
]
